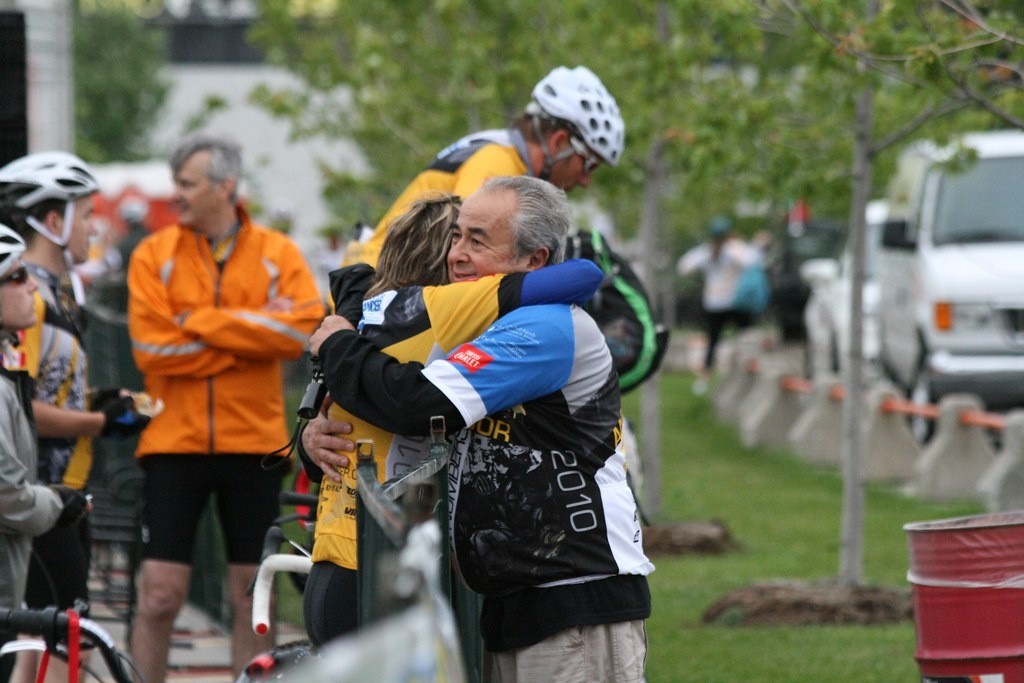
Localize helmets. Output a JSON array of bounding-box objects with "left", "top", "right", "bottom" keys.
[
  {"left": 1, "top": 151, "right": 99, "bottom": 232},
  {"left": 0, "top": 223, "right": 28, "bottom": 283},
  {"left": 524, "top": 65, "right": 626, "bottom": 169}
]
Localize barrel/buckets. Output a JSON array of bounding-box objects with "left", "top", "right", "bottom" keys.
[{"left": 903, "top": 509, "right": 1024, "bottom": 683}]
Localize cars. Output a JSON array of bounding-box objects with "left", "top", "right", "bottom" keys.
[{"left": 794, "top": 196, "right": 880, "bottom": 376}]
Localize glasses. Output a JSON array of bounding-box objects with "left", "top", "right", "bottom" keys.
[
  {"left": 0, "top": 265, "right": 29, "bottom": 285},
  {"left": 569, "top": 132, "right": 599, "bottom": 173}
]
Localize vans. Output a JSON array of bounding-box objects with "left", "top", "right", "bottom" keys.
[{"left": 868, "top": 127, "right": 1023, "bottom": 446}]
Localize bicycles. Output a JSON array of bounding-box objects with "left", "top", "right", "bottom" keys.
[{"left": 0, "top": 463, "right": 330, "bottom": 682}]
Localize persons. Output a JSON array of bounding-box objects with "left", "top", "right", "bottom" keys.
[
  {"left": 671, "top": 211, "right": 770, "bottom": 368},
  {"left": 125, "top": 134, "right": 327, "bottom": 683},
  {"left": 260, "top": 59, "right": 662, "bottom": 682},
  {"left": 0, "top": 148, "right": 153, "bottom": 682}
]
